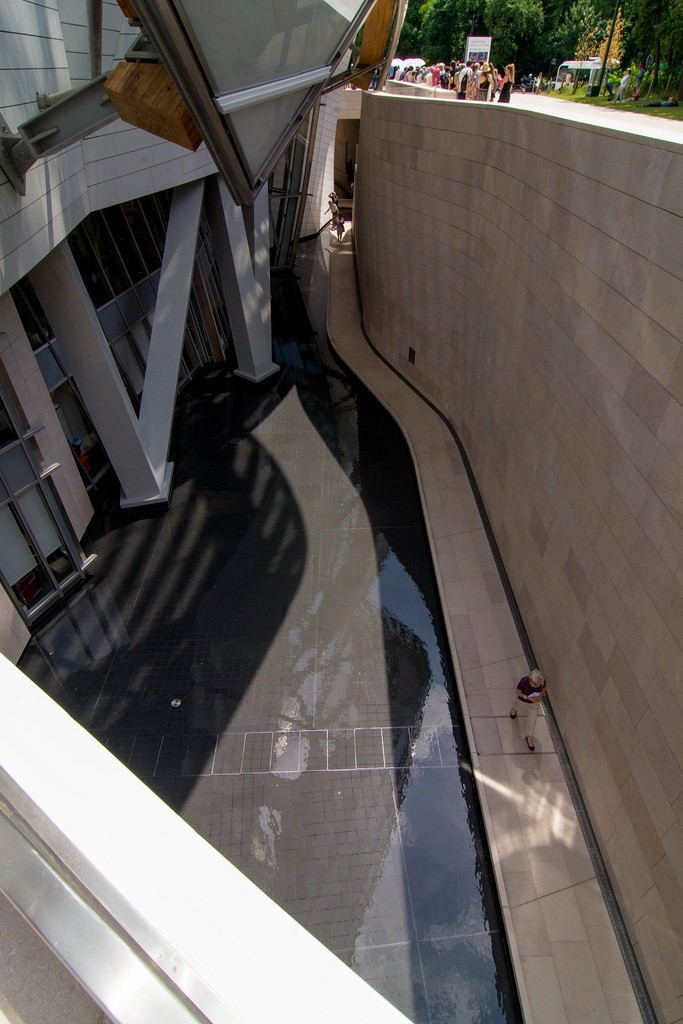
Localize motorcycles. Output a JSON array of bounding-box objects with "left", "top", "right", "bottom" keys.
[{"left": 520, "top": 75, "right": 537, "bottom": 91}]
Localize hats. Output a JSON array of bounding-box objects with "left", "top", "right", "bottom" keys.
[{"left": 480, "top": 62, "right": 490, "bottom": 72}]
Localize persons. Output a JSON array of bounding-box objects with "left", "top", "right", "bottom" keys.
[
  {"left": 510, "top": 670, "right": 547, "bottom": 751},
  {"left": 613, "top": 62, "right": 645, "bottom": 104},
  {"left": 324, "top": 191, "right": 345, "bottom": 243},
  {"left": 388, "top": 61, "right": 552, "bottom": 103},
  {"left": 644, "top": 97, "right": 678, "bottom": 107}
]
